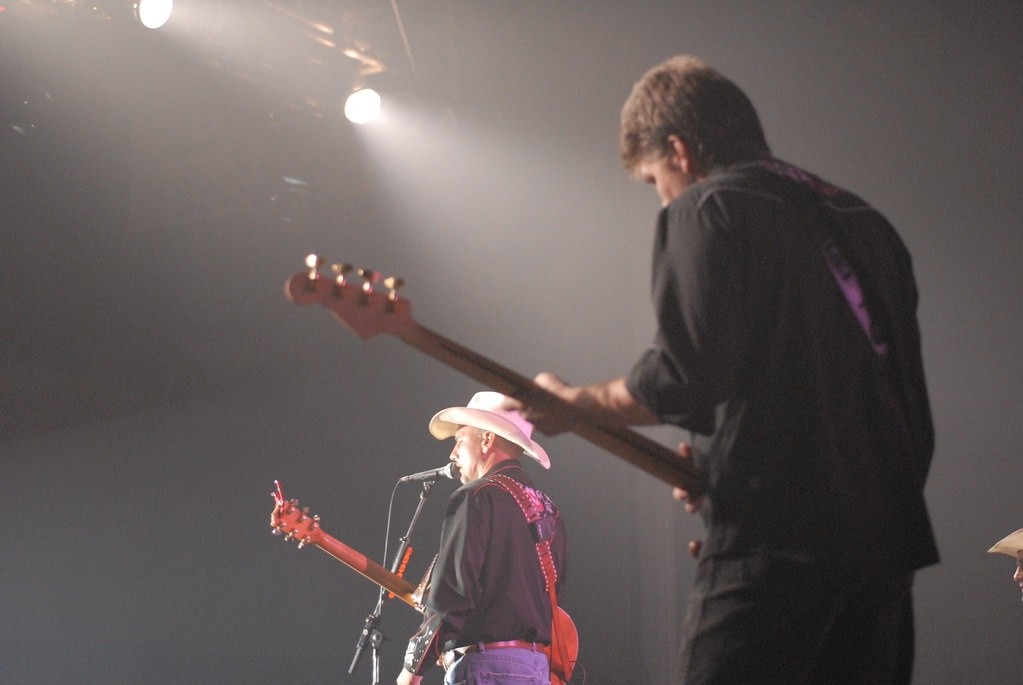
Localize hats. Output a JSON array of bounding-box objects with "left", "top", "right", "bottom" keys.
[{"left": 429, "top": 392, "right": 550, "bottom": 469}]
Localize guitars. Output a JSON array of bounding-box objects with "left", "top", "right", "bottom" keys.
[
  {"left": 282, "top": 253, "right": 709, "bottom": 498},
  {"left": 268, "top": 478, "right": 579, "bottom": 685}
]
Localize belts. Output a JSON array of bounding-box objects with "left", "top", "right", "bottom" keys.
[{"left": 466, "top": 640, "right": 546, "bottom": 653}]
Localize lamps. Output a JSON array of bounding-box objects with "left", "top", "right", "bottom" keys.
[
  {"left": 339, "top": 63, "right": 384, "bottom": 126},
  {"left": 91, "top": 0, "right": 176, "bottom": 31}
]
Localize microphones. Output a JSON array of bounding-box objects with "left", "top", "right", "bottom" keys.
[{"left": 399, "top": 462, "right": 461, "bottom": 484}]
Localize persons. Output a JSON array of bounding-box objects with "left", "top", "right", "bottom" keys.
[
  {"left": 502, "top": 56, "right": 940, "bottom": 685},
  {"left": 397, "top": 392, "right": 567, "bottom": 685}
]
